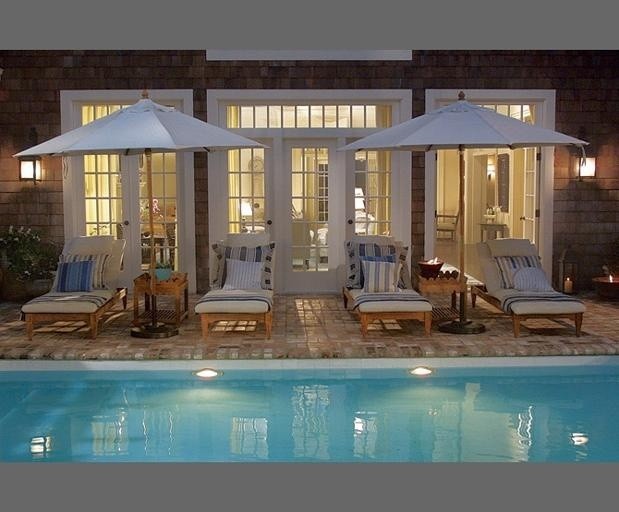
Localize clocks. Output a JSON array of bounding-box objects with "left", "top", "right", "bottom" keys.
[{"left": 248, "top": 155, "right": 265, "bottom": 177}]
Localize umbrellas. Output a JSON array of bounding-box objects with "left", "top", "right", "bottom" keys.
[
  {"left": 335, "top": 99, "right": 591, "bottom": 336},
  {"left": 9, "top": 98, "right": 271, "bottom": 338}
]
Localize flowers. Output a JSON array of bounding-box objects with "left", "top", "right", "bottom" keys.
[{"left": 142, "top": 196, "right": 161, "bottom": 216}]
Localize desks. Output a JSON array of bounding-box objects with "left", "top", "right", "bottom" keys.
[
  {"left": 412, "top": 270, "right": 468, "bottom": 322},
  {"left": 132, "top": 270, "right": 190, "bottom": 328},
  {"left": 480, "top": 221, "right": 507, "bottom": 244},
  {"left": 140, "top": 236, "right": 166, "bottom": 264}
]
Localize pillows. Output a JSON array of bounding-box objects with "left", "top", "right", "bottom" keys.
[
  {"left": 510, "top": 267, "right": 553, "bottom": 292},
  {"left": 52, "top": 257, "right": 96, "bottom": 294},
  {"left": 343, "top": 241, "right": 413, "bottom": 291},
  {"left": 221, "top": 257, "right": 266, "bottom": 291},
  {"left": 494, "top": 255, "right": 549, "bottom": 290},
  {"left": 211, "top": 239, "right": 277, "bottom": 290},
  {"left": 55, "top": 253, "right": 114, "bottom": 292},
  {"left": 360, "top": 255, "right": 405, "bottom": 290},
  {"left": 361, "top": 260, "right": 403, "bottom": 293}
]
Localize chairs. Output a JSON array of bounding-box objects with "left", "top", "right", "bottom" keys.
[
  {"left": 434, "top": 212, "right": 460, "bottom": 239},
  {"left": 286, "top": 217, "right": 314, "bottom": 270},
  {"left": 195, "top": 232, "right": 279, "bottom": 335},
  {"left": 466, "top": 239, "right": 583, "bottom": 339},
  {"left": 18, "top": 231, "right": 129, "bottom": 340},
  {"left": 342, "top": 234, "right": 435, "bottom": 337},
  {"left": 316, "top": 211, "right": 377, "bottom": 262}
]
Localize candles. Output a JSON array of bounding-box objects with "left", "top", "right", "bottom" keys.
[{"left": 563, "top": 277, "right": 575, "bottom": 293}]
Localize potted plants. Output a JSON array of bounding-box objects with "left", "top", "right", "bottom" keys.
[
  {"left": 3, "top": 223, "right": 38, "bottom": 301},
  {"left": 17, "top": 235, "right": 54, "bottom": 292}
]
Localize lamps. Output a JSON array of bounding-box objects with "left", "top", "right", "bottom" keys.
[
  {"left": 16, "top": 127, "right": 44, "bottom": 185},
  {"left": 571, "top": 139, "right": 597, "bottom": 184},
  {"left": 238, "top": 199, "right": 255, "bottom": 224},
  {"left": 28, "top": 431, "right": 54, "bottom": 461},
  {"left": 568, "top": 429, "right": 590, "bottom": 450}
]
